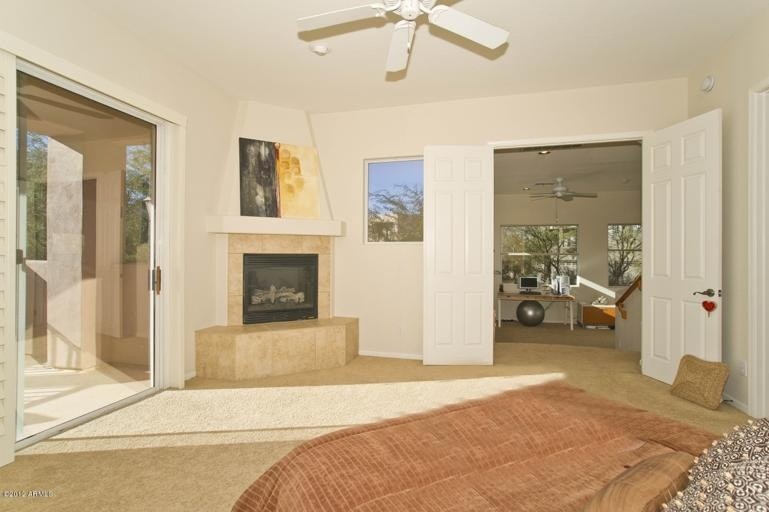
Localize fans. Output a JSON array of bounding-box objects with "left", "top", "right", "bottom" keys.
[{"left": 297, "top": 1, "right": 510, "bottom": 73}]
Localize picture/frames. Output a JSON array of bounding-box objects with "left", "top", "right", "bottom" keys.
[{"left": 363, "top": 155, "right": 426, "bottom": 245}]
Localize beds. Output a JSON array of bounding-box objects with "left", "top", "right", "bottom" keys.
[{"left": 256, "top": 381, "right": 769, "bottom": 512}]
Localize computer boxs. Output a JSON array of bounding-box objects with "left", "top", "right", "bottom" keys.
[{"left": 555, "top": 276, "right": 570, "bottom": 296}]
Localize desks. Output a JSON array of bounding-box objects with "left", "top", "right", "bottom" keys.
[{"left": 495, "top": 292, "right": 576, "bottom": 330}]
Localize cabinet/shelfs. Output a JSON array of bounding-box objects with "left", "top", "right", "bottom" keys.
[{"left": 578, "top": 303, "right": 616, "bottom": 329}]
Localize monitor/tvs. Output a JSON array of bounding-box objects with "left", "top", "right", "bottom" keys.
[{"left": 520, "top": 277, "right": 539, "bottom": 291}]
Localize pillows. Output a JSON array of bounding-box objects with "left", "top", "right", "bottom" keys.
[{"left": 586, "top": 414, "right": 769, "bottom": 512}]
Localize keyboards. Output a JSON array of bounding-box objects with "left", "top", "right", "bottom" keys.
[{"left": 519, "top": 291, "right": 542, "bottom": 295}]
